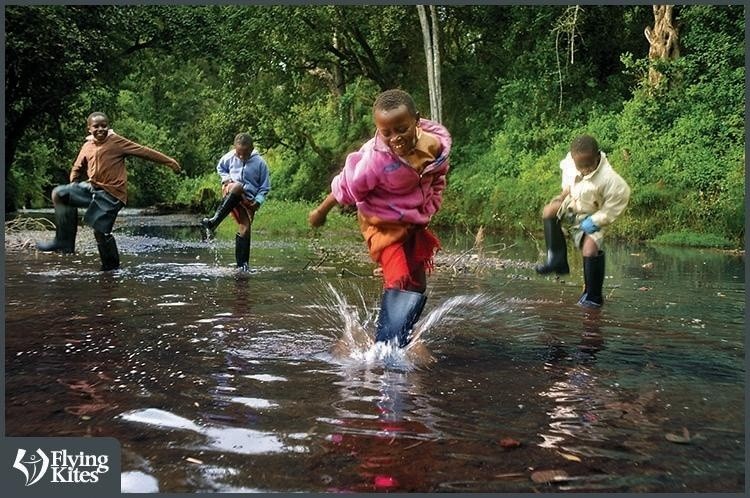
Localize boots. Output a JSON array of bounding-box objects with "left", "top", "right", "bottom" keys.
[
  {"left": 97, "top": 234, "right": 120, "bottom": 271},
  {"left": 202, "top": 192, "right": 241, "bottom": 233},
  {"left": 236, "top": 232, "right": 250, "bottom": 271},
  {"left": 576, "top": 249, "right": 605, "bottom": 307},
  {"left": 37, "top": 208, "right": 78, "bottom": 253},
  {"left": 373, "top": 287, "right": 428, "bottom": 372},
  {"left": 536, "top": 216, "right": 569, "bottom": 275}
]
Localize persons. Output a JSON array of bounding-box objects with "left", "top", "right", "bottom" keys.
[
  {"left": 536, "top": 133, "right": 631, "bottom": 310},
  {"left": 35, "top": 112, "right": 181, "bottom": 273},
  {"left": 309, "top": 89, "right": 452, "bottom": 373},
  {"left": 201, "top": 133, "right": 270, "bottom": 275}
]
